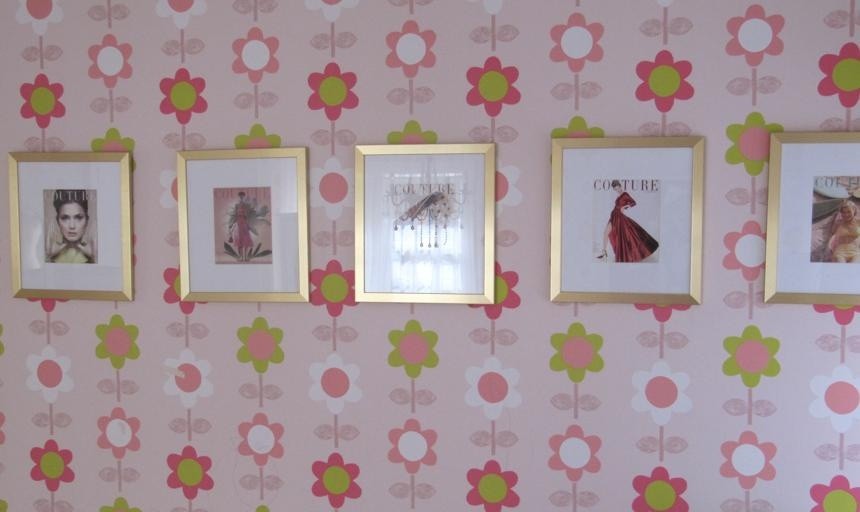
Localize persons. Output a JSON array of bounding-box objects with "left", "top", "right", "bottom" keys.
[
  {"left": 826, "top": 199, "right": 860, "bottom": 263},
  {"left": 45, "top": 189, "right": 96, "bottom": 264},
  {"left": 227, "top": 191, "right": 254, "bottom": 262},
  {"left": 596, "top": 179, "right": 660, "bottom": 264}
]
